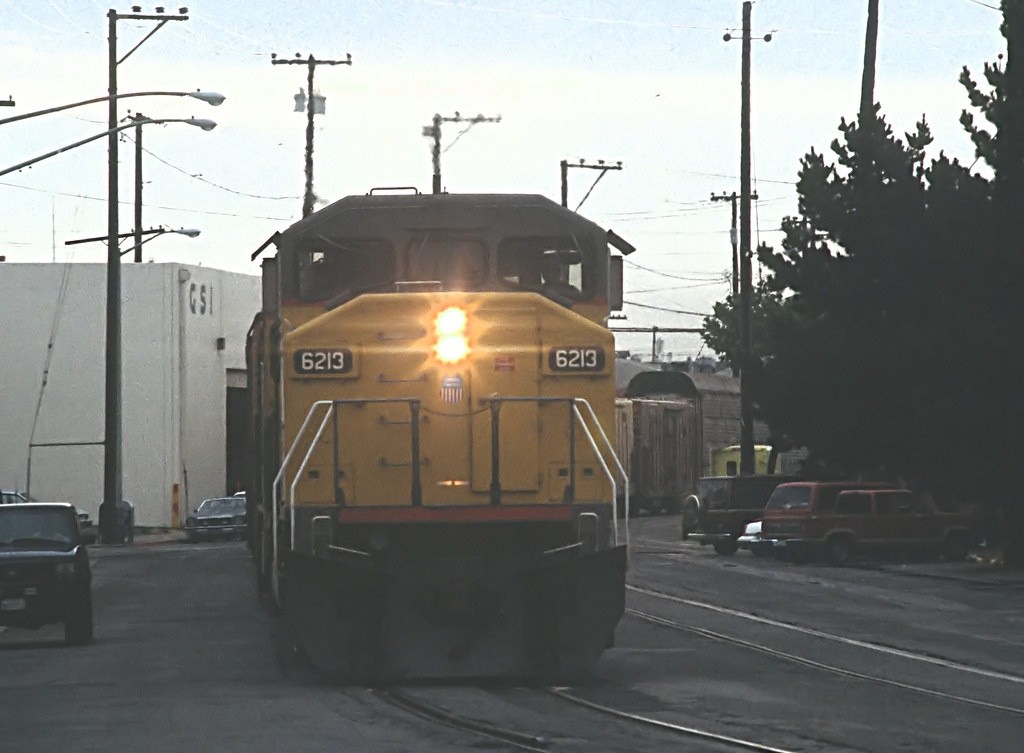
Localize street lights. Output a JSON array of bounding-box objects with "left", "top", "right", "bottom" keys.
[
  {"left": 65, "top": 226, "right": 201, "bottom": 543},
  {"left": 294, "top": 86, "right": 325, "bottom": 220}
]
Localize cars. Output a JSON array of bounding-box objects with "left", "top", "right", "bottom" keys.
[
  {"left": 0, "top": 491, "right": 94, "bottom": 641},
  {"left": 185, "top": 490, "right": 249, "bottom": 540}
]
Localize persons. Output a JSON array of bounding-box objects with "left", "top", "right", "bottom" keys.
[{"left": 543, "top": 265, "right": 581, "bottom": 297}]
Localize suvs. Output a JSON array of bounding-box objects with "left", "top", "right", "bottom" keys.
[{"left": 681, "top": 473, "right": 975, "bottom": 566}]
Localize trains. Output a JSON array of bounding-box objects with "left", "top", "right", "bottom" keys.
[{"left": 244, "top": 187, "right": 774, "bottom": 662}]
